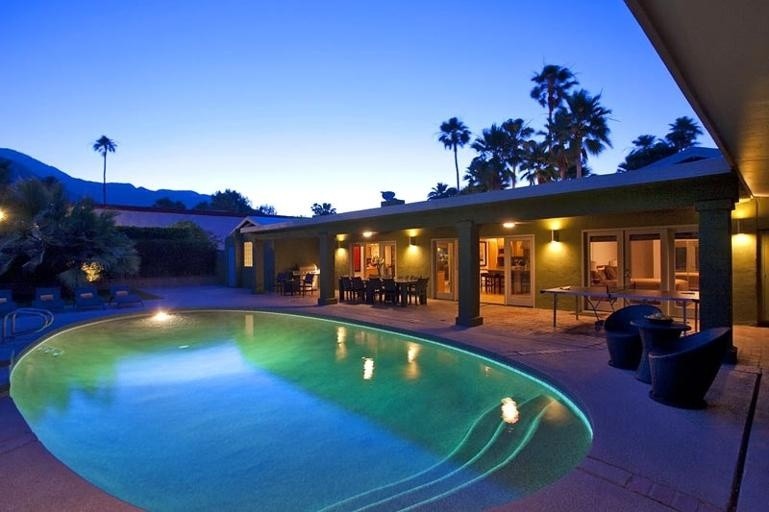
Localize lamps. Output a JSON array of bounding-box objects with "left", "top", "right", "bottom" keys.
[
  {"left": 409, "top": 237, "right": 417, "bottom": 245},
  {"left": 548, "top": 229, "right": 558, "bottom": 242},
  {"left": 335, "top": 241, "right": 345, "bottom": 248},
  {"left": 732, "top": 217, "right": 755, "bottom": 235}
]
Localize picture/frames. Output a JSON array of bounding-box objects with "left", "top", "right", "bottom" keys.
[{"left": 479, "top": 241, "right": 487, "bottom": 266}]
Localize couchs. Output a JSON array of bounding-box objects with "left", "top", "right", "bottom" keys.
[{"left": 600, "top": 279, "right": 689, "bottom": 291}]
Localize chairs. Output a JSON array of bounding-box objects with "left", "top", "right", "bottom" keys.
[
  {"left": 603, "top": 304, "right": 662, "bottom": 370},
  {"left": 339, "top": 275, "right": 430, "bottom": 308},
  {"left": 675, "top": 272, "right": 699, "bottom": 289},
  {"left": 276, "top": 272, "right": 315, "bottom": 298},
  {"left": 648, "top": 326, "right": 732, "bottom": 404}
]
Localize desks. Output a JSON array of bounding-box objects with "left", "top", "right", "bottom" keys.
[
  {"left": 540, "top": 286, "right": 700, "bottom": 335},
  {"left": 481, "top": 273, "right": 501, "bottom": 294},
  {"left": 630, "top": 320, "right": 691, "bottom": 386}
]
